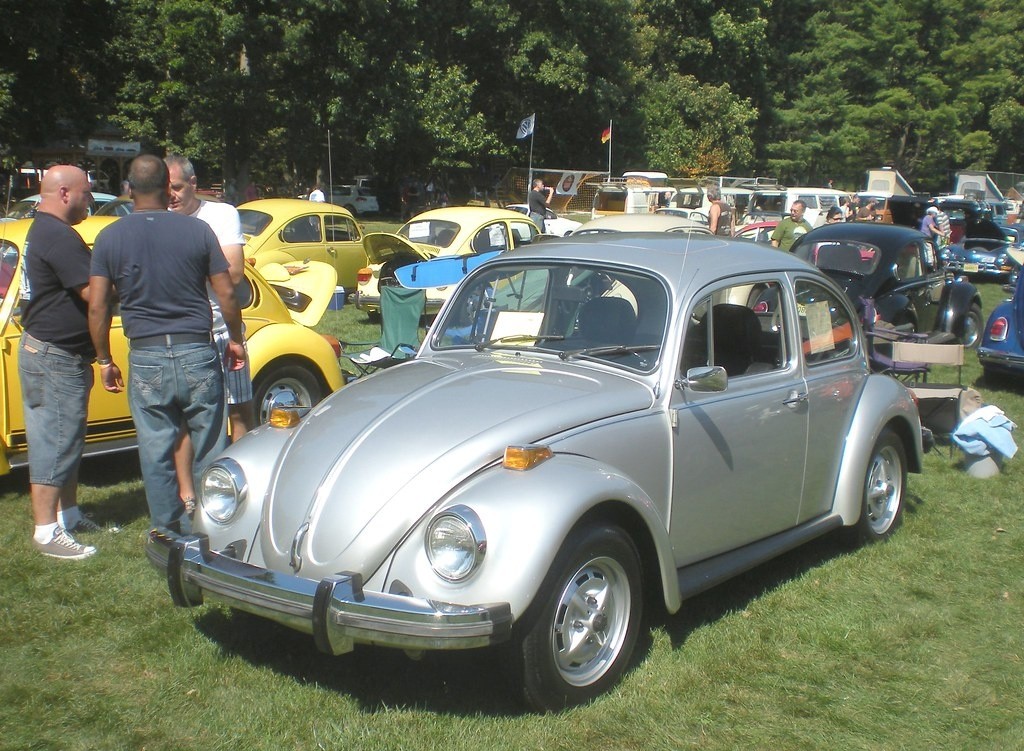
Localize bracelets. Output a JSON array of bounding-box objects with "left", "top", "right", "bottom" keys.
[{"left": 97, "top": 356, "right": 112, "bottom": 365}]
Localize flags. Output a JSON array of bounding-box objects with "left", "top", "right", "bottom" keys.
[
  {"left": 515, "top": 115, "right": 533, "bottom": 138},
  {"left": 601, "top": 126, "right": 612, "bottom": 143}
]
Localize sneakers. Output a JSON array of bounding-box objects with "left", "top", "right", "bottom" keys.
[
  {"left": 33, "top": 526, "right": 97, "bottom": 560},
  {"left": 64, "top": 513, "right": 123, "bottom": 534}
]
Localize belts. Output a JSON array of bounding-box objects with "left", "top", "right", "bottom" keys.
[
  {"left": 25, "top": 338, "right": 76, "bottom": 358},
  {"left": 130, "top": 333, "right": 210, "bottom": 345}
]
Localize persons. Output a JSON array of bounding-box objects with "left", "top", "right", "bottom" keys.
[
  {"left": 828, "top": 179, "right": 833, "bottom": 188},
  {"left": 660, "top": 192, "right": 671, "bottom": 207},
  {"left": 771, "top": 194, "right": 881, "bottom": 260},
  {"left": 527, "top": 179, "right": 553, "bottom": 241},
  {"left": 17, "top": 164, "right": 119, "bottom": 561},
  {"left": 88, "top": 155, "right": 247, "bottom": 541},
  {"left": 162, "top": 153, "right": 252, "bottom": 517},
  {"left": 305, "top": 184, "right": 325, "bottom": 233},
  {"left": 922, "top": 206, "right": 953, "bottom": 271},
  {"left": 707, "top": 185, "right": 734, "bottom": 237},
  {"left": 367, "top": 163, "right": 452, "bottom": 223}
]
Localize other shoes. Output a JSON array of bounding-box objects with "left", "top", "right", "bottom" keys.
[{"left": 183, "top": 496, "right": 197, "bottom": 518}]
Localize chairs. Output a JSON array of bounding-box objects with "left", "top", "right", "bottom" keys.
[
  {"left": 860, "top": 294, "right": 927, "bottom": 383},
  {"left": 891, "top": 342, "right": 972, "bottom": 460},
  {"left": 687, "top": 303, "right": 766, "bottom": 374},
  {"left": 337, "top": 286, "right": 426, "bottom": 381},
  {"left": 573, "top": 296, "right": 635, "bottom": 351}
]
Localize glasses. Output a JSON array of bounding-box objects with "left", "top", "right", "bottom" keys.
[
  {"left": 832, "top": 217, "right": 843, "bottom": 220},
  {"left": 790, "top": 209, "right": 798, "bottom": 213}
]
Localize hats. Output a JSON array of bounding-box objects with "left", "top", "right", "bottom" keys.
[
  {"left": 927, "top": 206, "right": 938, "bottom": 213},
  {"left": 869, "top": 198, "right": 879, "bottom": 203}
]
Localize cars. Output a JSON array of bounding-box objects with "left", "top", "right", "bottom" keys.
[
  {"left": 0, "top": 213, "right": 347, "bottom": 480},
  {"left": 586, "top": 172, "right": 1024, "bottom": 284},
  {"left": 750, "top": 221, "right": 983, "bottom": 349},
  {"left": 91, "top": 194, "right": 222, "bottom": 214},
  {"left": 235, "top": 198, "right": 373, "bottom": 288},
  {"left": 356, "top": 207, "right": 545, "bottom": 322},
  {"left": 144, "top": 228, "right": 935, "bottom": 716},
  {"left": 507, "top": 203, "right": 584, "bottom": 237},
  {"left": 976, "top": 269, "right": 1024, "bottom": 381},
  {"left": 564, "top": 212, "right": 773, "bottom": 324},
  {"left": 1, "top": 192, "right": 134, "bottom": 223}
]
